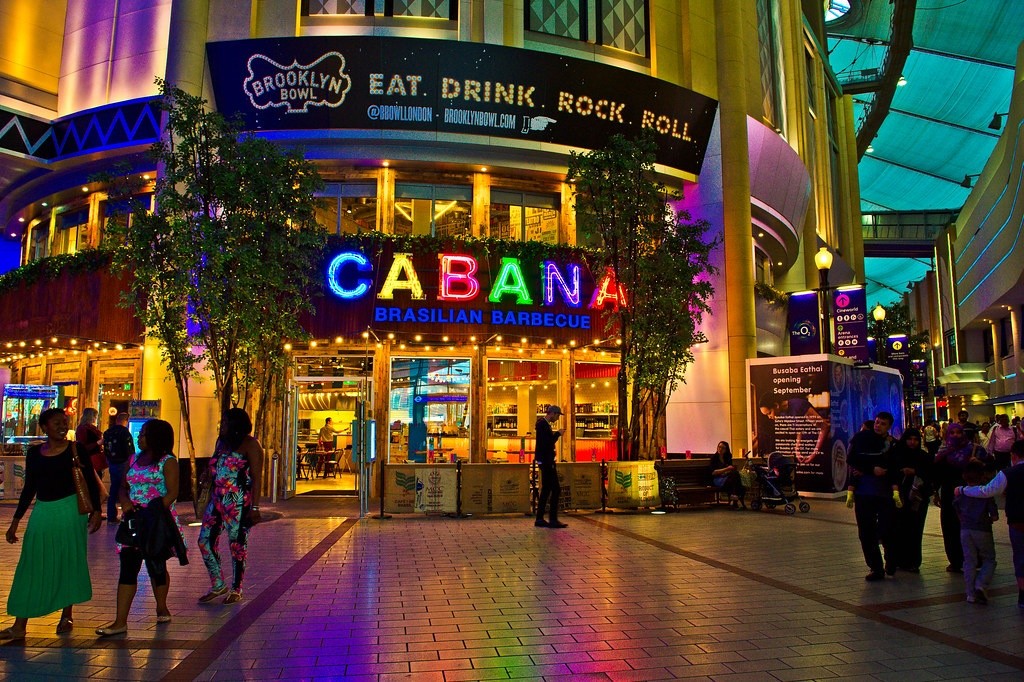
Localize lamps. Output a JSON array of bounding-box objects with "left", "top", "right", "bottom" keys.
[
  {"left": 988, "top": 112, "right": 1009, "bottom": 130},
  {"left": 347, "top": 206, "right": 352, "bottom": 214},
  {"left": 906, "top": 281, "right": 913, "bottom": 289},
  {"left": 898, "top": 74, "right": 906, "bottom": 86},
  {"left": 867, "top": 144, "right": 873, "bottom": 152},
  {"left": 961, "top": 174, "right": 981, "bottom": 188}
]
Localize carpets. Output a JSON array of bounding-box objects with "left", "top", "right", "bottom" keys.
[{"left": 298, "top": 490, "right": 360, "bottom": 495}]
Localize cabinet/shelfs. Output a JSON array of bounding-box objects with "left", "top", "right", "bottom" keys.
[{"left": 488, "top": 412, "right": 619, "bottom": 438}]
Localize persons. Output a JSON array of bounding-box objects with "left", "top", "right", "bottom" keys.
[
  {"left": 103, "top": 412, "right": 136, "bottom": 522},
  {"left": 532, "top": 405, "right": 569, "bottom": 529},
  {"left": 318, "top": 416, "right": 348, "bottom": 480},
  {"left": 758, "top": 391, "right": 828, "bottom": 466},
  {"left": 95, "top": 419, "right": 189, "bottom": 640},
  {"left": 846, "top": 409, "right": 1024, "bottom": 613},
  {"left": 76, "top": 408, "right": 109, "bottom": 521},
  {"left": 198, "top": 407, "right": 264, "bottom": 606},
  {"left": 0, "top": 407, "right": 103, "bottom": 641},
  {"left": 709, "top": 441, "right": 740, "bottom": 512},
  {"left": 951, "top": 462, "right": 999, "bottom": 607}
]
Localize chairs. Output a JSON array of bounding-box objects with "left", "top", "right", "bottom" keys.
[{"left": 297, "top": 442, "right": 353, "bottom": 481}]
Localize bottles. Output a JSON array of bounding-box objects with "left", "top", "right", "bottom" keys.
[
  {"left": 550, "top": 421, "right": 557, "bottom": 430},
  {"left": 495, "top": 416, "right": 517, "bottom": 429},
  {"left": 591, "top": 450, "right": 596, "bottom": 462},
  {"left": 575, "top": 399, "right": 617, "bottom": 414},
  {"left": 488, "top": 404, "right": 517, "bottom": 414},
  {"left": 451, "top": 450, "right": 457, "bottom": 463},
  {"left": 537, "top": 404, "right": 551, "bottom": 414},
  {"left": 519, "top": 448, "right": 525, "bottom": 464},
  {"left": 575, "top": 416, "right": 616, "bottom": 429}
]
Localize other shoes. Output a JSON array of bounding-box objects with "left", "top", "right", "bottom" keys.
[
  {"left": 885, "top": 567, "right": 895, "bottom": 575},
  {"left": 100, "top": 513, "right": 107, "bottom": 521},
  {"left": 534, "top": 519, "right": 551, "bottom": 528},
  {"left": 95, "top": 624, "right": 128, "bottom": 635},
  {"left": 157, "top": 609, "right": 171, "bottom": 622},
  {"left": 966, "top": 595, "right": 976, "bottom": 602},
  {"left": 975, "top": 586, "right": 989, "bottom": 600},
  {"left": 946, "top": 565, "right": 964, "bottom": 572},
  {"left": 549, "top": 520, "right": 568, "bottom": 528},
  {"left": 107, "top": 518, "right": 120, "bottom": 526},
  {"left": 1015, "top": 603, "right": 1024, "bottom": 611},
  {"left": 976, "top": 563, "right": 982, "bottom": 571},
  {"left": 866, "top": 571, "right": 885, "bottom": 581}
]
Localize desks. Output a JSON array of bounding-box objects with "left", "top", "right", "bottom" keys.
[
  {"left": 433, "top": 448, "right": 454, "bottom": 463},
  {"left": 507, "top": 449, "right": 535, "bottom": 454},
  {"left": 467, "top": 448, "right": 500, "bottom": 453}
]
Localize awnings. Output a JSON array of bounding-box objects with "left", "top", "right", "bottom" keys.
[{"left": 985, "top": 393, "right": 1024, "bottom": 405}]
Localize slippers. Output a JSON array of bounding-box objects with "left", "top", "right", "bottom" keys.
[
  {"left": 0, "top": 627, "right": 26, "bottom": 639},
  {"left": 56, "top": 616, "right": 73, "bottom": 634}
]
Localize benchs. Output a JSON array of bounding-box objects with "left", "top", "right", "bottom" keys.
[{"left": 654, "top": 459, "right": 746, "bottom": 512}]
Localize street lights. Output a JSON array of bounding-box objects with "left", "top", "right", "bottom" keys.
[
  {"left": 814, "top": 244, "right": 833, "bottom": 354},
  {"left": 873, "top": 303, "right": 885, "bottom": 367}
]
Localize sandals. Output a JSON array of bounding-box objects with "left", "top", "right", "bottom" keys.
[
  {"left": 224, "top": 588, "right": 243, "bottom": 606},
  {"left": 198, "top": 586, "right": 228, "bottom": 603}
]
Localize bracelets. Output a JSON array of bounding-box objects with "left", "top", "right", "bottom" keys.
[{"left": 251, "top": 505, "right": 259, "bottom": 511}]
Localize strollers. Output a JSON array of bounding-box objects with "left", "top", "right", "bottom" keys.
[{"left": 744, "top": 451, "right": 810, "bottom": 515}]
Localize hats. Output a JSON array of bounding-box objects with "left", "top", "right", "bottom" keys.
[{"left": 548, "top": 405, "right": 564, "bottom": 415}]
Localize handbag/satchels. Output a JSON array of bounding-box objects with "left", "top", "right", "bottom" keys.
[
  {"left": 739, "top": 460, "right": 752, "bottom": 487},
  {"left": 71, "top": 441, "right": 109, "bottom": 514},
  {"left": 114, "top": 506, "right": 146, "bottom": 548},
  {"left": 90, "top": 451, "right": 109, "bottom": 471},
  {"left": 196, "top": 471, "right": 215, "bottom": 517}
]
